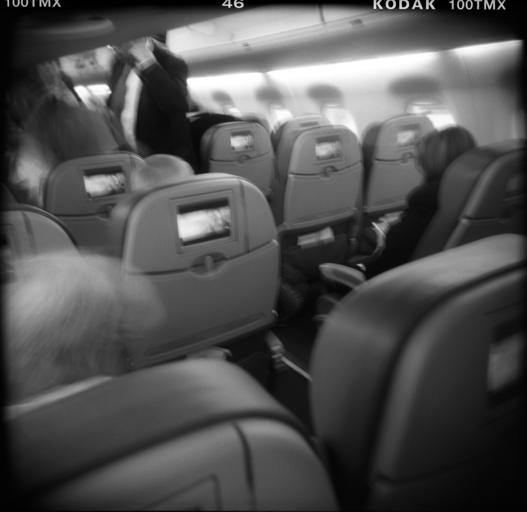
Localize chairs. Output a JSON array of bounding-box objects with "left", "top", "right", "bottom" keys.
[{"left": 0, "top": 110, "right": 527, "bottom": 512}]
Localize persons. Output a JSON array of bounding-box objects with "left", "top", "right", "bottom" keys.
[
  {"left": 323, "top": 126, "right": 477, "bottom": 300},
  {"left": 29, "top": 58, "right": 86, "bottom": 107},
  {"left": 106, "top": 34, "right": 190, "bottom": 161},
  {"left": 1, "top": 249, "right": 167, "bottom": 405}
]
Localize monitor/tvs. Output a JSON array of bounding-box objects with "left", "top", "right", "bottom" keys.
[
  {"left": 82, "top": 167, "right": 127, "bottom": 200},
  {"left": 393, "top": 125, "right": 419, "bottom": 146},
  {"left": 228, "top": 129, "right": 256, "bottom": 155},
  {"left": 174, "top": 195, "right": 235, "bottom": 247},
  {"left": 314, "top": 135, "right": 344, "bottom": 162}
]
